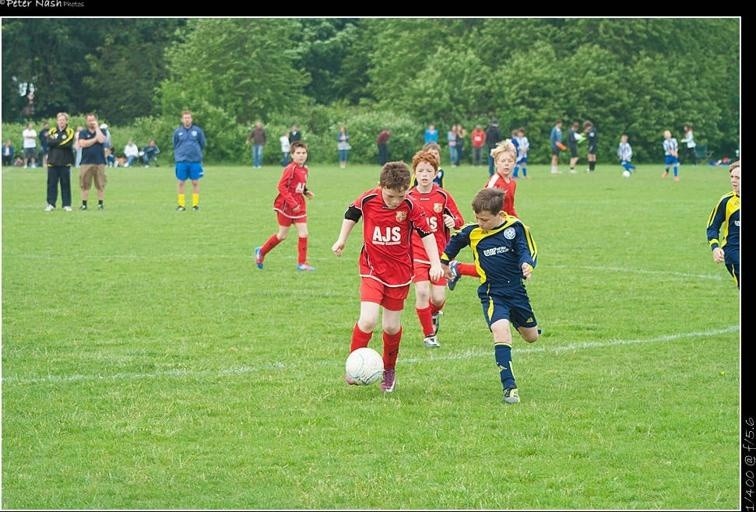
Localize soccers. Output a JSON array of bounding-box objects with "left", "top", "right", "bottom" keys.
[
  {"left": 346, "top": 347, "right": 384, "bottom": 385},
  {"left": 623, "top": 170, "right": 631, "bottom": 177}
]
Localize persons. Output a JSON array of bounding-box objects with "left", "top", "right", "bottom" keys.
[
  {"left": 446, "top": 139, "right": 518, "bottom": 292},
  {"left": 408, "top": 140, "right": 445, "bottom": 190},
  {"left": 707, "top": 156, "right": 735, "bottom": 166},
  {"left": 4, "top": 118, "right": 600, "bottom": 174},
  {"left": 661, "top": 129, "right": 681, "bottom": 183},
  {"left": 171, "top": 111, "right": 210, "bottom": 213},
  {"left": 331, "top": 160, "right": 446, "bottom": 393},
  {"left": 439, "top": 186, "right": 541, "bottom": 405},
  {"left": 76, "top": 111, "right": 111, "bottom": 210},
  {"left": 703, "top": 161, "right": 740, "bottom": 289},
  {"left": 251, "top": 141, "right": 317, "bottom": 272},
  {"left": 616, "top": 134, "right": 636, "bottom": 176},
  {"left": 406, "top": 150, "right": 465, "bottom": 349},
  {"left": 679, "top": 121, "right": 701, "bottom": 167},
  {"left": 42, "top": 110, "right": 73, "bottom": 214}
]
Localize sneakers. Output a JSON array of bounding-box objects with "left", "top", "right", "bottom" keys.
[
  {"left": 44, "top": 204, "right": 199, "bottom": 212},
  {"left": 296, "top": 264, "right": 314, "bottom": 271},
  {"left": 381, "top": 260, "right": 520, "bottom": 404},
  {"left": 255, "top": 247, "right": 264, "bottom": 269}
]
